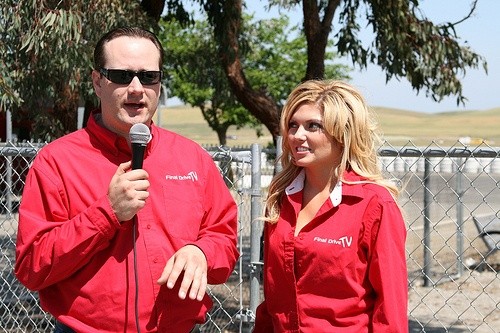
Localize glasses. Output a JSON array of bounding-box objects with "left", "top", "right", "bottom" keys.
[{"left": 98, "top": 66, "right": 165, "bottom": 86}]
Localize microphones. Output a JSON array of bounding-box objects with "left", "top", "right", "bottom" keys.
[{"left": 129, "top": 123, "right": 150, "bottom": 171}]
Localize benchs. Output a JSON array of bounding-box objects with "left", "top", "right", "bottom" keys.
[{"left": 469, "top": 214, "right": 500, "bottom": 273}]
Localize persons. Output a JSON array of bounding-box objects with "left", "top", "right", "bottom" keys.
[
  {"left": 251, "top": 79, "right": 409, "bottom": 333},
  {"left": 13, "top": 26, "right": 240, "bottom": 333}
]
